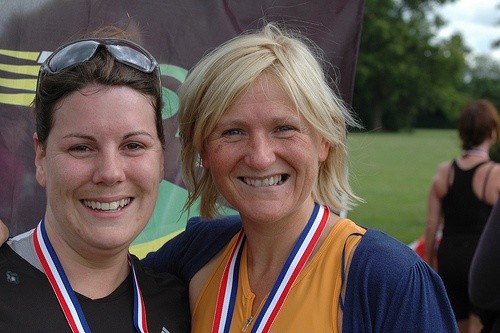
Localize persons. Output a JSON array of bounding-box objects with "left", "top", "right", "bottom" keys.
[
  {"left": 0, "top": 22, "right": 459, "bottom": 333},
  {"left": 0, "top": 26, "right": 192, "bottom": 333},
  {"left": 425, "top": 99, "right": 500, "bottom": 333}
]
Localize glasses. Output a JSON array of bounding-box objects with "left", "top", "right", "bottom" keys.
[{"left": 33, "top": 37, "right": 162, "bottom": 100}]
